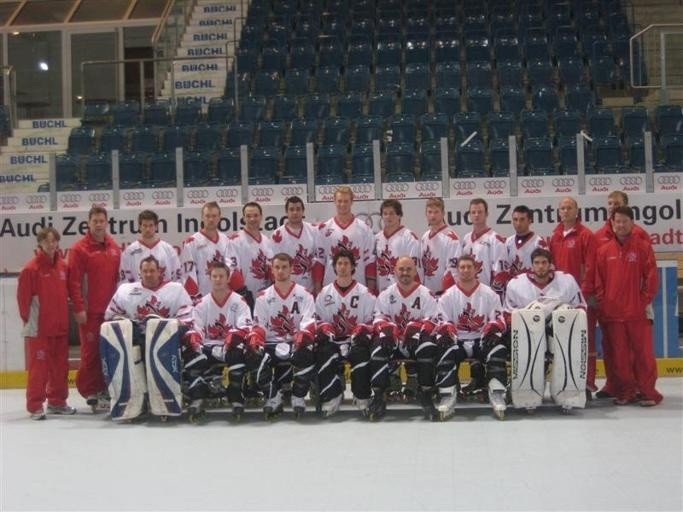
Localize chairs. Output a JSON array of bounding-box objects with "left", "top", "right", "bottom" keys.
[{"left": 55, "top": 2, "right": 683, "bottom": 192}]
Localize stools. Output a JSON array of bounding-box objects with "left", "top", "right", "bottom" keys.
[{"left": 58, "top": 353, "right": 603, "bottom": 417}]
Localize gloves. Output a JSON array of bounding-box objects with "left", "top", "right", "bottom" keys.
[
  {"left": 352, "top": 331, "right": 370, "bottom": 347},
  {"left": 402, "top": 327, "right": 423, "bottom": 352},
  {"left": 436, "top": 335, "right": 453, "bottom": 350},
  {"left": 379, "top": 331, "right": 396, "bottom": 351},
  {"left": 316, "top": 333, "right": 330, "bottom": 347},
  {"left": 247, "top": 346, "right": 266, "bottom": 365},
  {"left": 223, "top": 347, "right": 244, "bottom": 363},
  {"left": 480, "top": 328, "right": 502, "bottom": 348},
  {"left": 291, "top": 349, "right": 314, "bottom": 366},
  {"left": 186, "top": 350, "right": 208, "bottom": 368}
]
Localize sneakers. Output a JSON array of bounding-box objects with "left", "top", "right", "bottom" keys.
[
  {"left": 45, "top": 403, "right": 77, "bottom": 416},
  {"left": 588, "top": 390, "right": 661, "bottom": 406},
  {"left": 189, "top": 400, "right": 204, "bottom": 415},
  {"left": 264, "top": 398, "right": 283, "bottom": 414},
  {"left": 488, "top": 392, "right": 508, "bottom": 412},
  {"left": 29, "top": 408, "right": 45, "bottom": 421},
  {"left": 232, "top": 403, "right": 246, "bottom": 416},
  {"left": 419, "top": 396, "right": 457, "bottom": 418},
  {"left": 292, "top": 396, "right": 305, "bottom": 411},
  {"left": 321, "top": 395, "right": 341, "bottom": 412},
  {"left": 355, "top": 398, "right": 387, "bottom": 418},
  {"left": 86, "top": 394, "right": 111, "bottom": 410}
]
